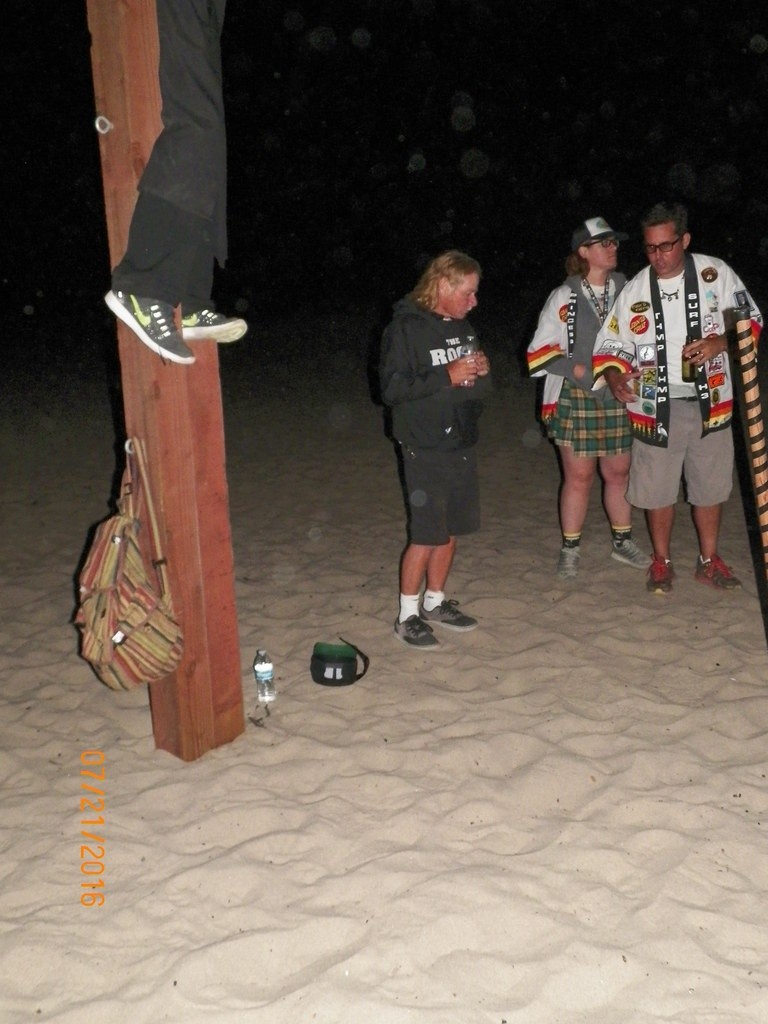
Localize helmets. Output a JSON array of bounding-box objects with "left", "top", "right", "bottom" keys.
[{"left": 310, "top": 642, "right": 359, "bottom": 687}]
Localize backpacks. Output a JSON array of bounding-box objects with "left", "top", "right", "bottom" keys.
[{"left": 72, "top": 437, "right": 184, "bottom": 690}]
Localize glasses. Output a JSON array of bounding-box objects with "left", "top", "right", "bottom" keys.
[
  {"left": 339, "top": 636, "right": 369, "bottom": 681},
  {"left": 584, "top": 238, "right": 620, "bottom": 247},
  {"left": 642, "top": 235, "right": 684, "bottom": 254}
]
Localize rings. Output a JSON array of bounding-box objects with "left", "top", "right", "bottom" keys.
[{"left": 700, "top": 353, "right": 703, "bottom": 357}]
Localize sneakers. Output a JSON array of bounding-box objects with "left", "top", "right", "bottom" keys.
[
  {"left": 611, "top": 540, "right": 653, "bottom": 569},
  {"left": 557, "top": 546, "right": 581, "bottom": 586},
  {"left": 419, "top": 599, "right": 478, "bottom": 632},
  {"left": 393, "top": 614, "right": 439, "bottom": 649},
  {"left": 695, "top": 554, "right": 742, "bottom": 592},
  {"left": 645, "top": 553, "right": 676, "bottom": 594},
  {"left": 181, "top": 308, "right": 248, "bottom": 343},
  {"left": 105, "top": 289, "right": 195, "bottom": 365}
]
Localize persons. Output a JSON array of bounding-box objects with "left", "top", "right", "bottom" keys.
[
  {"left": 102, "top": 0, "right": 249, "bottom": 365},
  {"left": 377, "top": 250, "right": 490, "bottom": 650},
  {"left": 590, "top": 202, "right": 764, "bottom": 596},
  {"left": 527, "top": 218, "right": 653, "bottom": 578}
]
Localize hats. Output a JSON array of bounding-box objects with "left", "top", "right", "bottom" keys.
[{"left": 571, "top": 216, "right": 629, "bottom": 251}]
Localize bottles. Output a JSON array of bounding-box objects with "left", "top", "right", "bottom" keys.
[
  {"left": 254, "top": 649, "right": 275, "bottom": 702},
  {"left": 682, "top": 335, "right": 698, "bottom": 383},
  {"left": 457, "top": 336, "right": 477, "bottom": 386}
]
